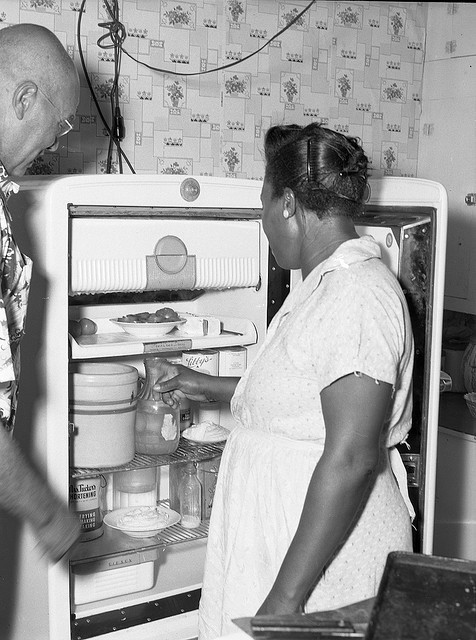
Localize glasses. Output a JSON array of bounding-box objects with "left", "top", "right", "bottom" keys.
[{"left": 6, "top": 80, "right": 77, "bottom": 137}]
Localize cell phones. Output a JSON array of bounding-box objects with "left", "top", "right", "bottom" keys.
[{"left": 250, "top": 614, "right": 356, "bottom": 632}]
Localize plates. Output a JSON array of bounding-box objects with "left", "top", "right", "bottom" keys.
[
  {"left": 105, "top": 313, "right": 187, "bottom": 338},
  {"left": 103, "top": 506, "right": 182, "bottom": 538}
]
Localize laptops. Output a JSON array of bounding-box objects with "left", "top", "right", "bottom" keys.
[{"left": 364, "top": 549, "right": 475, "bottom": 640}]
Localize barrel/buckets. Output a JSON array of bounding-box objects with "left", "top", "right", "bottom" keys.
[{"left": 73, "top": 362, "right": 141, "bottom": 468}]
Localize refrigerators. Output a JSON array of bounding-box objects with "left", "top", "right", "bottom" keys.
[{"left": 0, "top": 169, "right": 451, "bottom": 640}]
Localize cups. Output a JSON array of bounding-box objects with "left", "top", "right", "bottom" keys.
[{"left": 112, "top": 460, "right": 159, "bottom": 513}]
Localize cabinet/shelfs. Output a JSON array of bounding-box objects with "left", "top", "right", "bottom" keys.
[{"left": 414, "top": 0, "right": 476, "bottom": 317}]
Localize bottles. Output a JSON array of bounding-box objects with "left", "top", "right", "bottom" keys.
[
  {"left": 177, "top": 459, "right": 204, "bottom": 528},
  {"left": 130, "top": 355, "right": 182, "bottom": 458}
]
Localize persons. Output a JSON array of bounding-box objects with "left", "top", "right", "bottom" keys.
[
  {"left": 0, "top": 24, "right": 81, "bottom": 564},
  {"left": 151, "top": 122, "right": 418, "bottom": 640}
]
